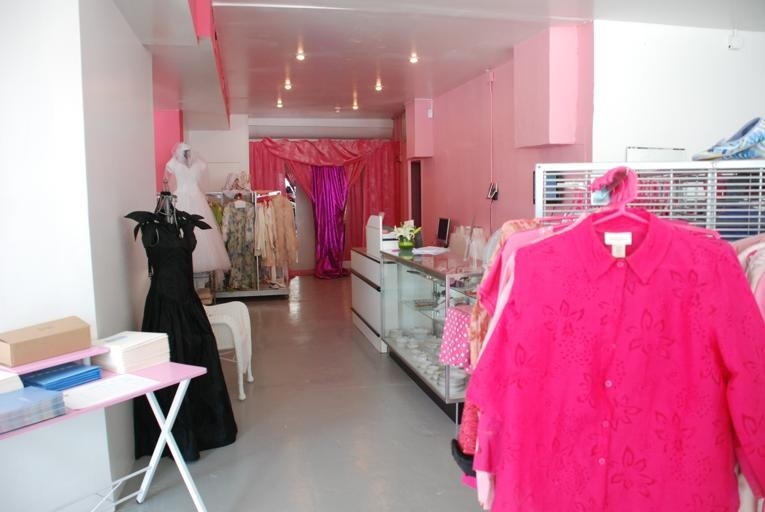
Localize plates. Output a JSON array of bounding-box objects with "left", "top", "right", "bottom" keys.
[{"left": 389, "top": 325, "right": 470, "bottom": 392}]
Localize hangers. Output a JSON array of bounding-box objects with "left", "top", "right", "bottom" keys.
[
  {"left": 499, "top": 163, "right": 721, "bottom": 265},
  {"left": 208, "top": 189, "right": 297, "bottom": 209},
  {"left": 731, "top": 231, "right": 764, "bottom": 267}
]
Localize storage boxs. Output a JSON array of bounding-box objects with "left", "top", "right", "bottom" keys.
[{"left": 0, "top": 316, "right": 90, "bottom": 367}]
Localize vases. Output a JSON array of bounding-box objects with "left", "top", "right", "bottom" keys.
[{"left": 399, "top": 235, "right": 416, "bottom": 257}]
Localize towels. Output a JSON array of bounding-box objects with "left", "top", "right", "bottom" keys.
[{"left": 411, "top": 246, "right": 449, "bottom": 256}]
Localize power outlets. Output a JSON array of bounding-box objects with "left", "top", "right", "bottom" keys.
[{"left": 491, "top": 188, "right": 499, "bottom": 201}]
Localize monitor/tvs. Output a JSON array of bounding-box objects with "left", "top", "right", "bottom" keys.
[{"left": 436, "top": 217, "right": 450, "bottom": 247}]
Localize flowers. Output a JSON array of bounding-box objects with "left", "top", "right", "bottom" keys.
[{"left": 391, "top": 222, "right": 421, "bottom": 242}]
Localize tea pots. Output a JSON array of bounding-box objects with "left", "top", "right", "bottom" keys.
[{"left": 448, "top": 224, "right": 487, "bottom": 273}]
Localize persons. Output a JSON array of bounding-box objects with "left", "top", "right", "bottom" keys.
[
  {"left": 123, "top": 189, "right": 238, "bottom": 462},
  {"left": 164, "top": 142, "right": 233, "bottom": 274}
]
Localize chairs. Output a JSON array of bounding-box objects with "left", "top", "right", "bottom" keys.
[{"left": 203, "top": 300, "right": 255, "bottom": 402}]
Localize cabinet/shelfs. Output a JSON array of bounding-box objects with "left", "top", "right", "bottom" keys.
[
  {"left": 379, "top": 247, "right": 488, "bottom": 425},
  {"left": 351, "top": 250, "right": 380, "bottom": 337}
]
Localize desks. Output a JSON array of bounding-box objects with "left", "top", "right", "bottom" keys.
[
  {"left": 439, "top": 305, "right": 475, "bottom": 374},
  {"left": 0, "top": 343, "right": 208, "bottom": 512}
]
